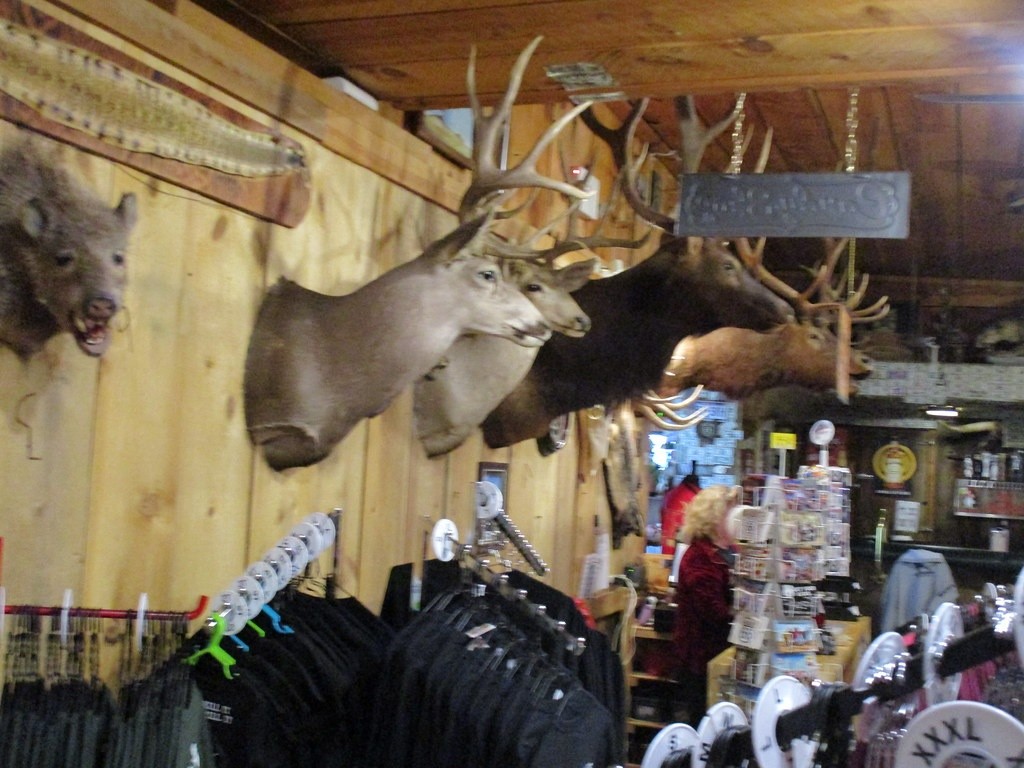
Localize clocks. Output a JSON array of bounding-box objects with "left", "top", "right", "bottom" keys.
[{"left": 701, "top": 423, "right": 716, "bottom": 437}]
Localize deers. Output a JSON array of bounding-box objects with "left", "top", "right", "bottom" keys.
[{"left": 243, "top": 36, "right": 891, "bottom": 474}]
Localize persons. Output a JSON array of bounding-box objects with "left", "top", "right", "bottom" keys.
[
  {"left": 673, "top": 484, "right": 734, "bottom": 725},
  {"left": 662, "top": 474, "right": 702, "bottom": 554}
]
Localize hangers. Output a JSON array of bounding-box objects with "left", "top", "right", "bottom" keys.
[{"left": 4, "top": 508, "right": 1018, "bottom": 768}]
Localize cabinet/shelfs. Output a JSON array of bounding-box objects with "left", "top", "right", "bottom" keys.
[
  {"left": 619, "top": 624, "right": 683, "bottom": 768},
  {"left": 705, "top": 615, "right": 872, "bottom": 709}
]
